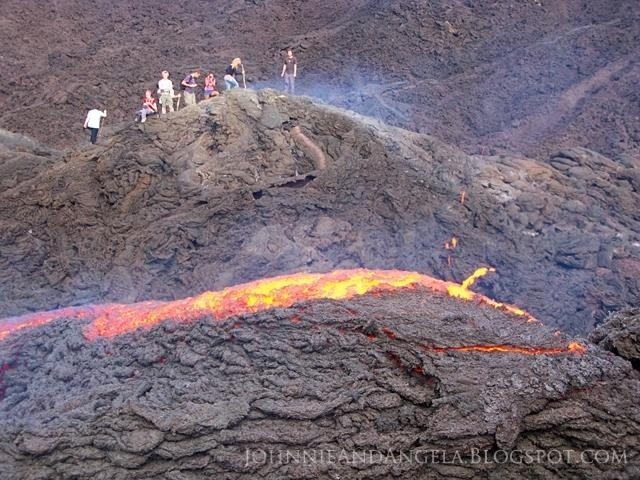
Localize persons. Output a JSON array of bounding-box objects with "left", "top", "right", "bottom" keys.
[
  {"left": 281, "top": 48, "right": 297, "bottom": 92},
  {"left": 83, "top": 105, "right": 107, "bottom": 144},
  {"left": 135, "top": 89, "right": 158, "bottom": 123},
  {"left": 157, "top": 70, "right": 181, "bottom": 115},
  {"left": 224, "top": 57, "right": 244, "bottom": 91},
  {"left": 203, "top": 72, "right": 219, "bottom": 100},
  {"left": 181, "top": 70, "right": 201, "bottom": 106}
]
